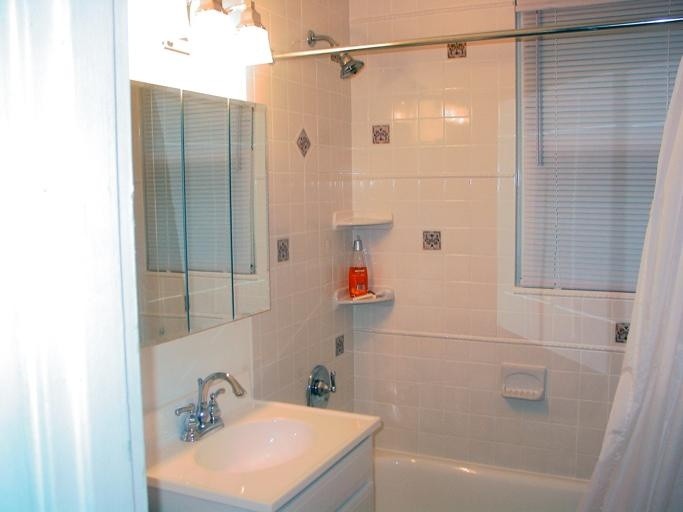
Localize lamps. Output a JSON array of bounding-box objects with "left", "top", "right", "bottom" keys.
[
  {"left": 161, "top": 1, "right": 275, "bottom": 73},
  {"left": 304, "top": 29, "right": 366, "bottom": 81}
]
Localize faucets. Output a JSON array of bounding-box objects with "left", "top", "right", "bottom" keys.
[{"left": 196, "top": 370, "right": 245, "bottom": 428}]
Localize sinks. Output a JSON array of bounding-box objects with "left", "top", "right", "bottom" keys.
[{"left": 195, "top": 414, "right": 318, "bottom": 470}]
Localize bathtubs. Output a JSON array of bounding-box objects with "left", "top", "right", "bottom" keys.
[{"left": 369, "top": 425, "right": 591, "bottom": 512}]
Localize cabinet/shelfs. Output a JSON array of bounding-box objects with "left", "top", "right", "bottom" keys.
[{"left": 328, "top": 211, "right": 397, "bottom": 308}]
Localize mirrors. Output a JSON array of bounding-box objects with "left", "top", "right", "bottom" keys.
[
  {"left": 229, "top": 98, "right": 274, "bottom": 321},
  {"left": 180, "top": 88, "right": 232, "bottom": 333},
  {"left": 130, "top": 77, "right": 187, "bottom": 345}
]
